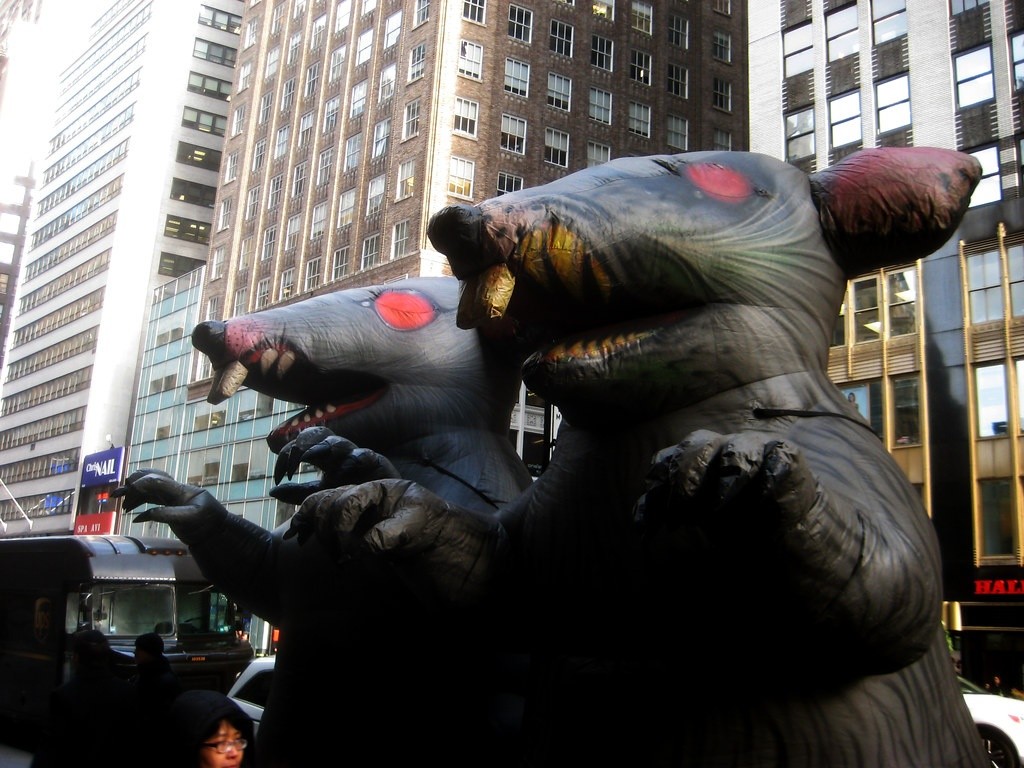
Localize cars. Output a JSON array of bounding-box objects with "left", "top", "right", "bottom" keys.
[
  {"left": 957, "top": 674, "right": 1024, "bottom": 768},
  {"left": 226, "top": 656, "right": 278, "bottom": 741}
]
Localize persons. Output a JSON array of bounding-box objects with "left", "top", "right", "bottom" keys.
[{"left": 29, "top": 629, "right": 255, "bottom": 768}]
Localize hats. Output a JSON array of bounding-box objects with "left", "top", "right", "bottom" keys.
[
  {"left": 228, "top": 621, "right": 246, "bottom": 631},
  {"left": 135, "top": 633, "right": 164, "bottom": 656}
]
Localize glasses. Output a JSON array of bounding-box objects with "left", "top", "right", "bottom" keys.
[{"left": 202, "top": 739, "right": 247, "bottom": 754}]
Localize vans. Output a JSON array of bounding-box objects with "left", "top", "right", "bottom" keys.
[{"left": 0, "top": 533, "right": 256, "bottom": 768}]
